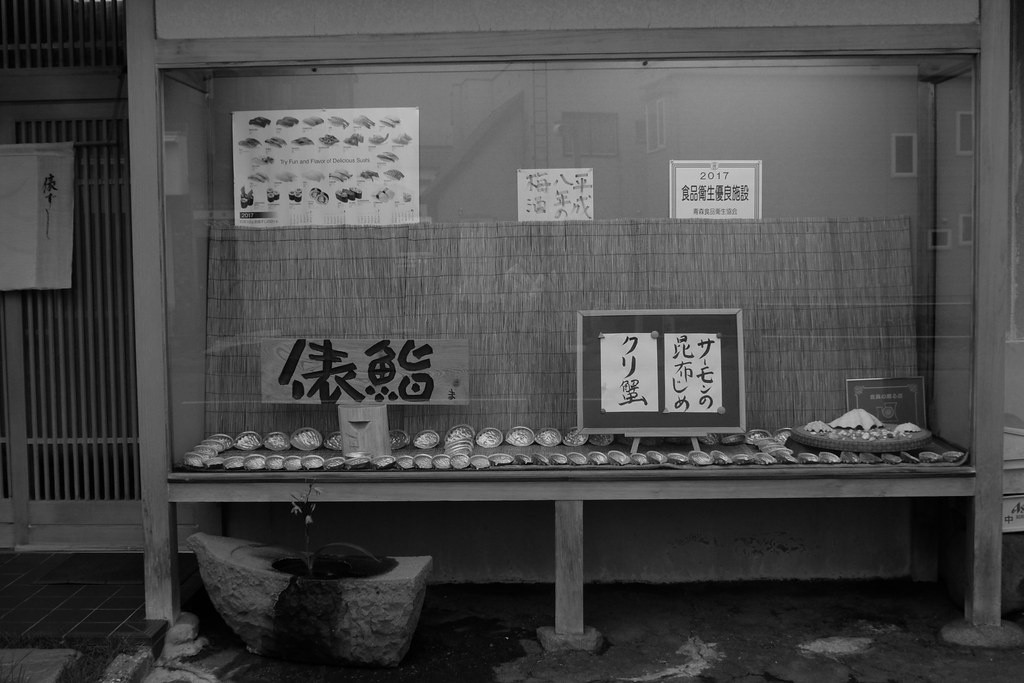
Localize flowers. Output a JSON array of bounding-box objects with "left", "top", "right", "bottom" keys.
[{"left": 281, "top": 471, "right": 380, "bottom": 576}]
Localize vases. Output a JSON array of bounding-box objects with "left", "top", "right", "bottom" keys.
[{"left": 186, "top": 531, "right": 433, "bottom": 668}]
